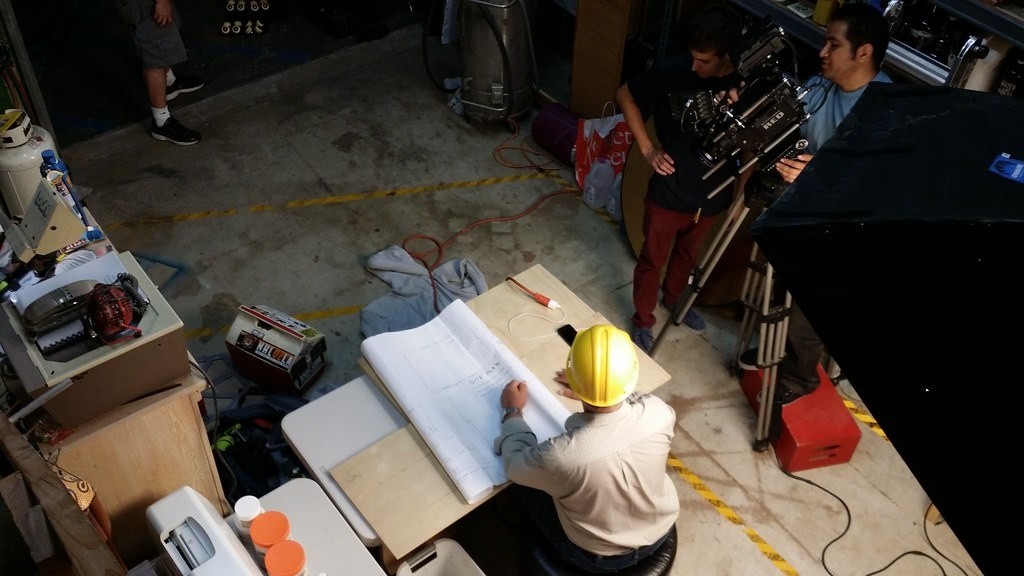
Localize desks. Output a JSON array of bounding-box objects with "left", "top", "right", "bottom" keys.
[{"left": 280, "top": 262, "right": 674, "bottom": 552}]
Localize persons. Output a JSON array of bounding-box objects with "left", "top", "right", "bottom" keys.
[
  {"left": 737, "top": 3, "right": 893, "bottom": 404},
  {"left": 616, "top": 4, "right": 756, "bottom": 355},
  {"left": 114, "top": 0, "right": 205, "bottom": 146},
  {"left": 494, "top": 324, "right": 680, "bottom": 576}
]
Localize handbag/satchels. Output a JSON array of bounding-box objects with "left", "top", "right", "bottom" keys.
[{"left": 576, "top": 99, "right": 638, "bottom": 191}]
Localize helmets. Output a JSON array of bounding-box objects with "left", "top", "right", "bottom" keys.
[{"left": 567, "top": 324, "right": 639, "bottom": 407}]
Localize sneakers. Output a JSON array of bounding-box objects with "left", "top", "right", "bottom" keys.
[
  {"left": 634, "top": 320, "right": 655, "bottom": 355},
  {"left": 661, "top": 298, "right": 705, "bottom": 334},
  {"left": 151, "top": 114, "right": 199, "bottom": 145},
  {"left": 164, "top": 73, "right": 204, "bottom": 100}
]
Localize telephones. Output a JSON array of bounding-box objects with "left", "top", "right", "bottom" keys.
[{"left": 23, "top": 280, "right": 98, "bottom": 338}]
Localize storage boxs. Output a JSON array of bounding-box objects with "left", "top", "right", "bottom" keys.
[{"left": 223, "top": 303, "right": 329, "bottom": 395}]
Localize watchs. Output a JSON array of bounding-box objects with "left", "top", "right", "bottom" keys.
[{"left": 498, "top": 407, "right": 522, "bottom": 424}]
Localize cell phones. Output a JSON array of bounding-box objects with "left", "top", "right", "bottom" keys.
[{"left": 558, "top": 324, "right": 577, "bottom": 347}]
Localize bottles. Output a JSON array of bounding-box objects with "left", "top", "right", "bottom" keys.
[
  {"left": 39, "top": 149, "right": 82, "bottom": 220},
  {"left": 264, "top": 539, "right": 306, "bottom": 576},
  {"left": 582, "top": 158, "right": 614, "bottom": 210},
  {"left": 234, "top": 495, "right": 266, "bottom": 549},
  {"left": 606, "top": 171, "right": 622, "bottom": 221},
  {"left": 250, "top": 511, "right": 292, "bottom": 567}
]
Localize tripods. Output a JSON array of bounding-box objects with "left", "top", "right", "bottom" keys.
[{"left": 647, "top": 130, "right": 812, "bottom": 452}]
